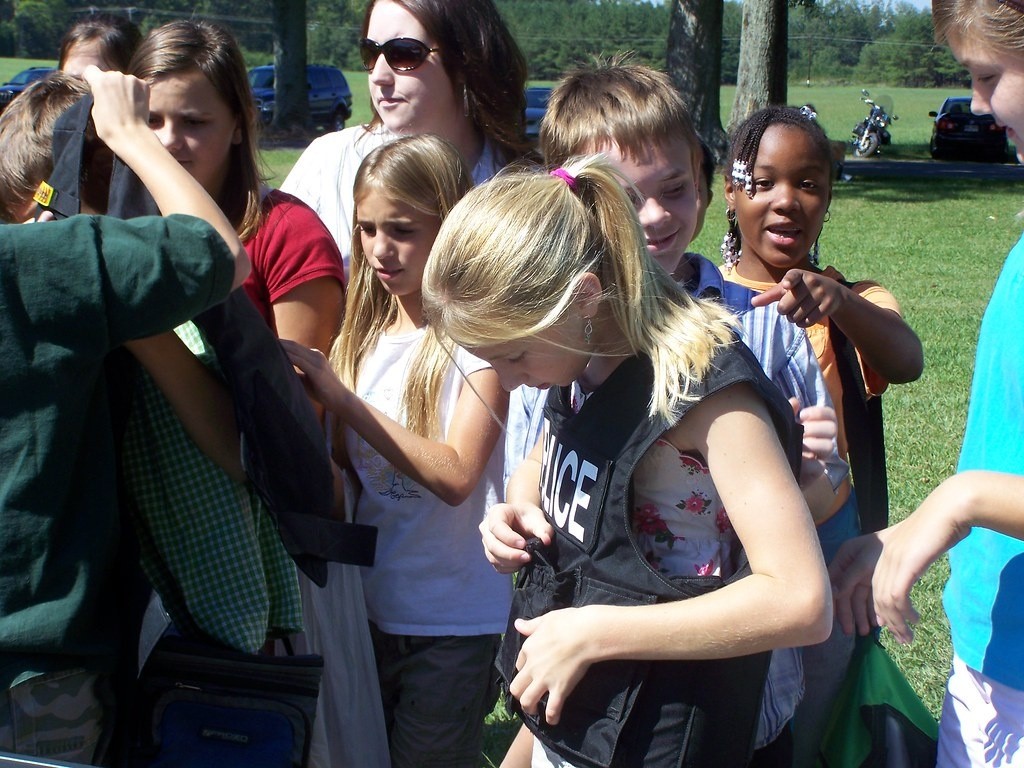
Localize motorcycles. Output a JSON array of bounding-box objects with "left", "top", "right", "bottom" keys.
[{"left": 851, "top": 88, "right": 898, "bottom": 158}]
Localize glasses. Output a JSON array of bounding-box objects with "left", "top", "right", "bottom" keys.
[{"left": 359, "top": 37, "right": 441, "bottom": 72}]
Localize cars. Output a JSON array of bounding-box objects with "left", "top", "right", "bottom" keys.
[
  {"left": 0, "top": 67, "right": 59, "bottom": 108},
  {"left": 927, "top": 97, "right": 1008, "bottom": 160},
  {"left": 248, "top": 63, "right": 352, "bottom": 132}
]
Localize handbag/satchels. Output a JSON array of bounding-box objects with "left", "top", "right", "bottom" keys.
[{"left": 106, "top": 624, "right": 324, "bottom": 768}]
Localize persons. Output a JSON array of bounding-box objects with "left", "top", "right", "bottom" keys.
[
  {"left": 0, "top": 0, "right": 923, "bottom": 768},
  {"left": 825, "top": 0, "right": 1024, "bottom": 768}
]
